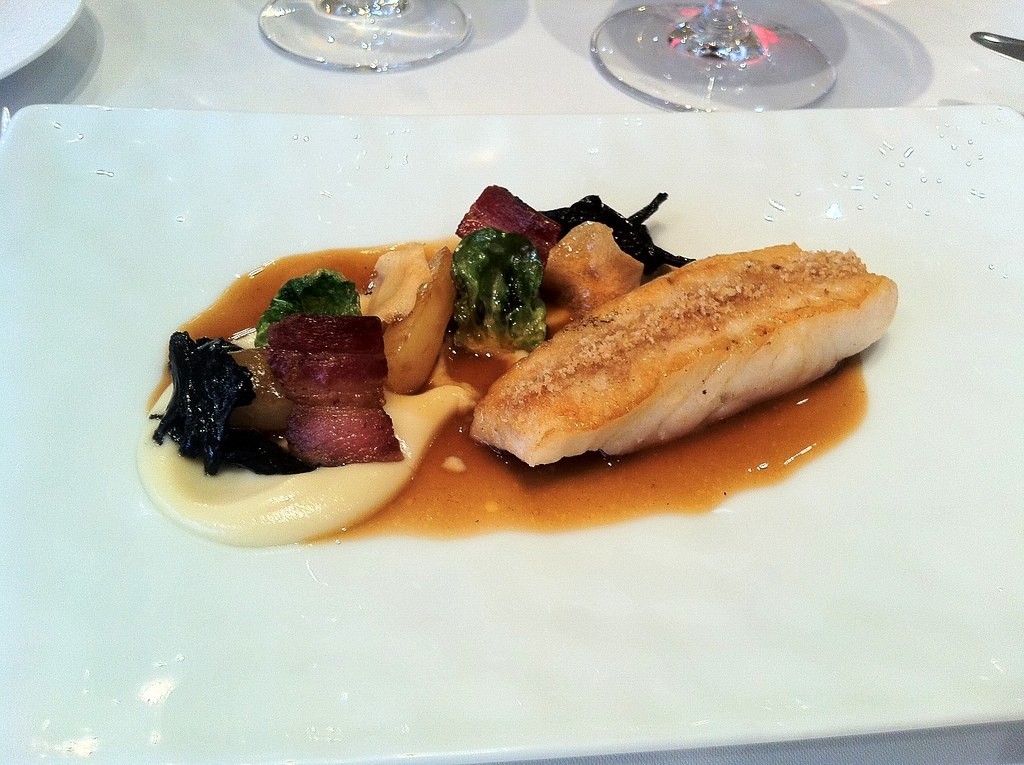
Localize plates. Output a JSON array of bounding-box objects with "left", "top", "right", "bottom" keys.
[{"left": 0, "top": 97, "right": 1024, "bottom": 765}]
